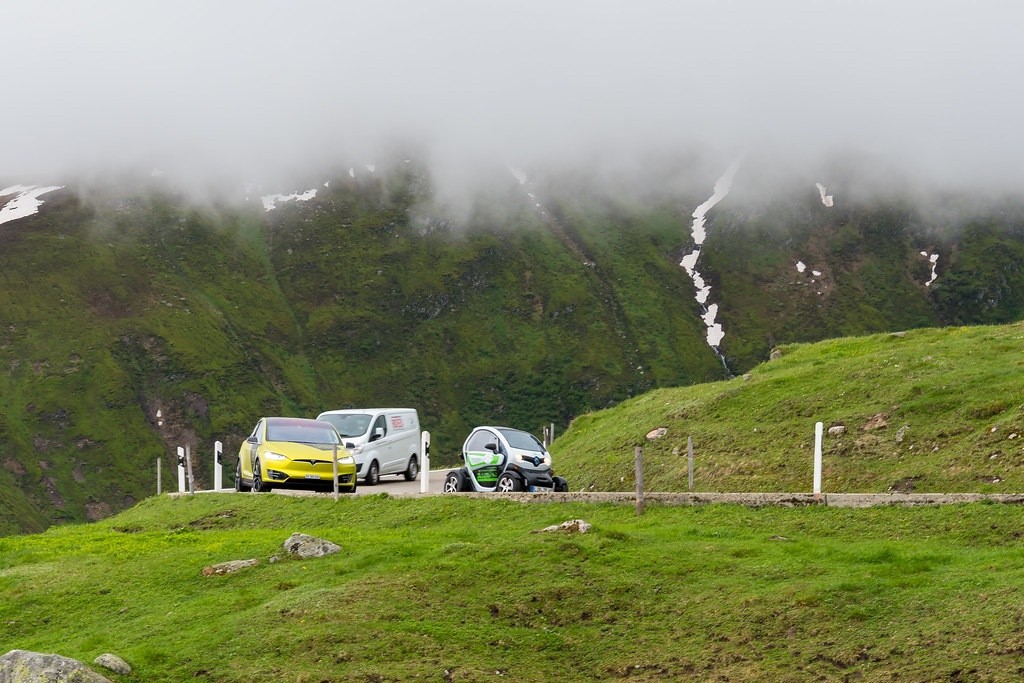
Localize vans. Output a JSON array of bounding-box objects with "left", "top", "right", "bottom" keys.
[{"left": 316, "top": 408, "right": 421, "bottom": 486}]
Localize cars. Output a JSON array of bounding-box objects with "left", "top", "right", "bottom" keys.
[
  {"left": 234, "top": 417, "right": 358, "bottom": 493},
  {"left": 444, "top": 426, "right": 568, "bottom": 492}
]
